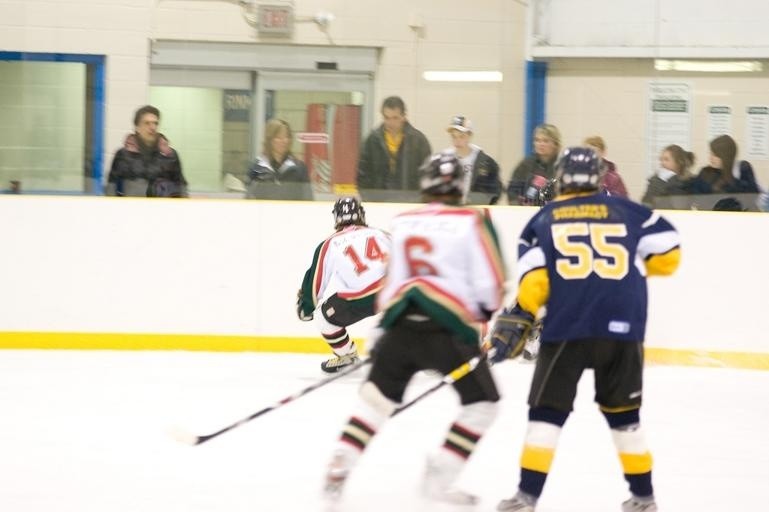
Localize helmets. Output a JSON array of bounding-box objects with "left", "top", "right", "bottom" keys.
[
  {"left": 558, "top": 147, "right": 600, "bottom": 192},
  {"left": 332, "top": 196, "right": 365, "bottom": 230},
  {"left": 419, "top": 153, "right": 463, "bottom": 198}
]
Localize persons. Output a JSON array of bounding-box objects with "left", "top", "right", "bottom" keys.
[
  {"left": 509, "top": 117, "right": 765, "bottom": 212},
  {"left": 106, "top": 105, "right": 190, "bottom": 198},
  {"left": 323, "top": 153, "right": 511, "bottom": 509},
  {"left": 352, "top": 96, "right": 430, "bottom": 202},
  {"left": 295, "top": 193, "right": 391, "bottom": 375},
  {"left": 489, "top": 143, "right": 680, "bottom": 510},
  {"left": 421, "top": 112, "right": 504, "bottom": 203},
  {"left": 243, "top": 117, "right": 311, "bottom": 200}
]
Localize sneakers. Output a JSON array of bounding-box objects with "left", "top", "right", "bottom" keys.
[
  {"left": 498, "top": 495, "right": 535, "bottom": 512},
  {"left": 322, "top": 356, "right": 362, "bottom": 375},
  {"left": 622, "top": 499, "right": 657, "bottom": 512}
]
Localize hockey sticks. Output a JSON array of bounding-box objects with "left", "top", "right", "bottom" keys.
[
  {"left": 162, "top": 360, "right": 370, "bottom": 447},
  {"left": 360, "top": 346, "right": 497, "bottom": 419}
]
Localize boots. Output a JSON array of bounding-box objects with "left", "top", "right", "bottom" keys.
[
  {"left": 422, "top": 451, "right": 479, "bottom": 506},
  {"left": 323, "top": 442, "right": 362, "bottom": 496}
]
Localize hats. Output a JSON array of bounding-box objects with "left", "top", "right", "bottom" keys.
[{"left": 445, "top": 114, "right": 474, "bottom": 133}]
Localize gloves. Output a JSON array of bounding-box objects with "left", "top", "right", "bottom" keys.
[{"left": 482, "top": 303, "right": 535, "bottom": 363}]
